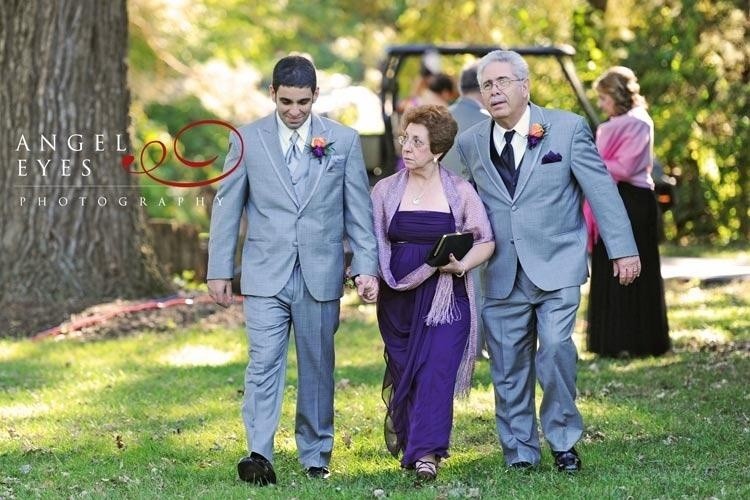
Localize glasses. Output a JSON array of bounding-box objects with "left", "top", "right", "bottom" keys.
[
  {"left": 480, "top": 78, "right": 524, "bottom": 95},
  {"left": 397, "top": 135, "right": 431, "bottom": 148}
]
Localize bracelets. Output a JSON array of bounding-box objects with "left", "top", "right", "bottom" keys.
[{"left": 456, "top": 261, "right": 466, "bottom": 278}]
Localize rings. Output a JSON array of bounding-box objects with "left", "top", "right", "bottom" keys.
[
  {"left": 634, "top": 270, "right": 637, "bottom": 273},
  {"left": 367, "top": 293, "right": 370, "bottom": 298}
]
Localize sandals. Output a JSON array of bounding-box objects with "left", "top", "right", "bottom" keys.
[{"left": 416, "top": 459, "right": 437, "bottom": 480}]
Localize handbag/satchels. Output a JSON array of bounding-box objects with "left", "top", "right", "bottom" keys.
[{"left": 425, "top": 231, "right": 474, "bottom": 267}]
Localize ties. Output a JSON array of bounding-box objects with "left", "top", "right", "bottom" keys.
[
  {"left": 501, "top": 130, "right": 516, "bottom": 177},
  {"left": 285, "top": 131, "right": 302, "bottom": 175}
]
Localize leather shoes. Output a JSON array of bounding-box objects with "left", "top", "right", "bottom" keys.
[
  {"left": 512, "top": 462, "right": 533, "bottom": 475},
  {"left": 306, "top": 466, "right": 329, "bottom": 480},
  {"left": 555, "top": 446, "right": 581, "bottom": 474},
  {"left": 238, "top": 456, "right": 276, "bottom": 487}
]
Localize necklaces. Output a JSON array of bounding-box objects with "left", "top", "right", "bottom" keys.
[{"left": 408, "top": 174, "right": 431, "bottom": 205}]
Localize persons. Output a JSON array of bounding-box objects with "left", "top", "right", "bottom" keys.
[
  {"left": 440, "top": 64, "right": 492, "bottom": 186},
  {"left": 580, "top": 66, "right": 673, "bottom": 358},
  {"left": 449, "top": 48, "right": 642, "bottom": 472},
  {"left": 420, "top": 52, "right": 445, "bottom": 82},
  {"left": 412, "top": 78, "right": 458, "bottom": 111},
  {"left": 206, "top": 54, "right": 381, "bottom": 484},
  {"left": 356, "top": 105, "right": 496, "bottom": 483}
]
